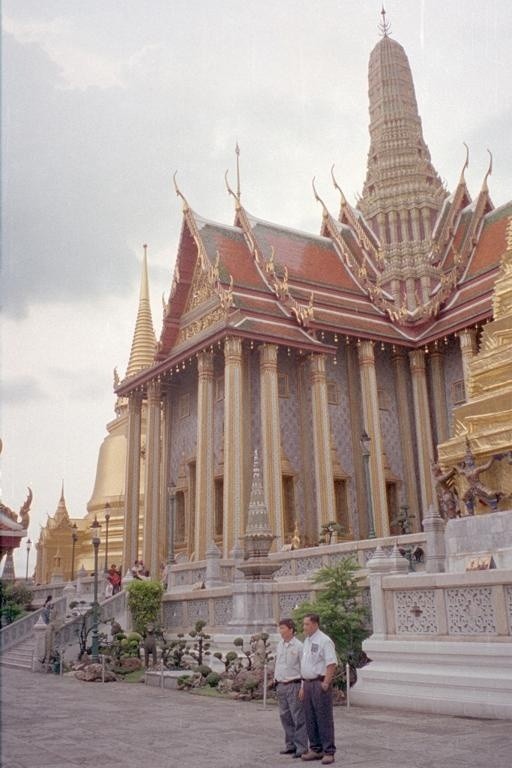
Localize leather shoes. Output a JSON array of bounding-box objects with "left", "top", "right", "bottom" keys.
[
  {"left": 280, "top": 748, "right": 296, "bottom": 753},
  {"left": 292, "top": 752, "right": 301, "bottom": 757},
  {"left": 301, "top": 750, "right": 324, "bottom": 760},
  {"left": 321, "top": 754, "right": 334, "bottom": 763}
]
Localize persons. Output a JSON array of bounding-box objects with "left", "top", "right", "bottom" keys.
[
  {"left": 299, "top": 612, "right": 339, "bottom": 765},
  {"left": 132, "top": 559, "right": 145, "bottom": 579},
  {"left": 430, "top": 463, "right": 464, "bottom": 520},
  {"left": 108, "top": 563, "right": 121, "bottom": 593},
  {"left": 103, "top": 577, "right": 114, "bottom": 599},
  {"left": 42, "top": 594, "right": 54, "bottom": 625},
  {"left": 271, "top": 617, "right": 313, "bottom": 759},
  {"left": 445, "top": 449, "right": 505, "bottom": 514}
]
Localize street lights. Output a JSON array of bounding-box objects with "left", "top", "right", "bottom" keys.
[
  {"left": 359, "top": 427, "right": 379, "bottom": 540},
  {"left": 89, "top": 513, "right": 102, "bottom": 664},
  {"left": 69, "top": 522, "right": 78, "bottom": 583},
  {"left": 24, "top": 537, "right": 33, "bottom": 585},
  {"left": 102, "top": 501, "right": 112, "bottom": 573}
]
